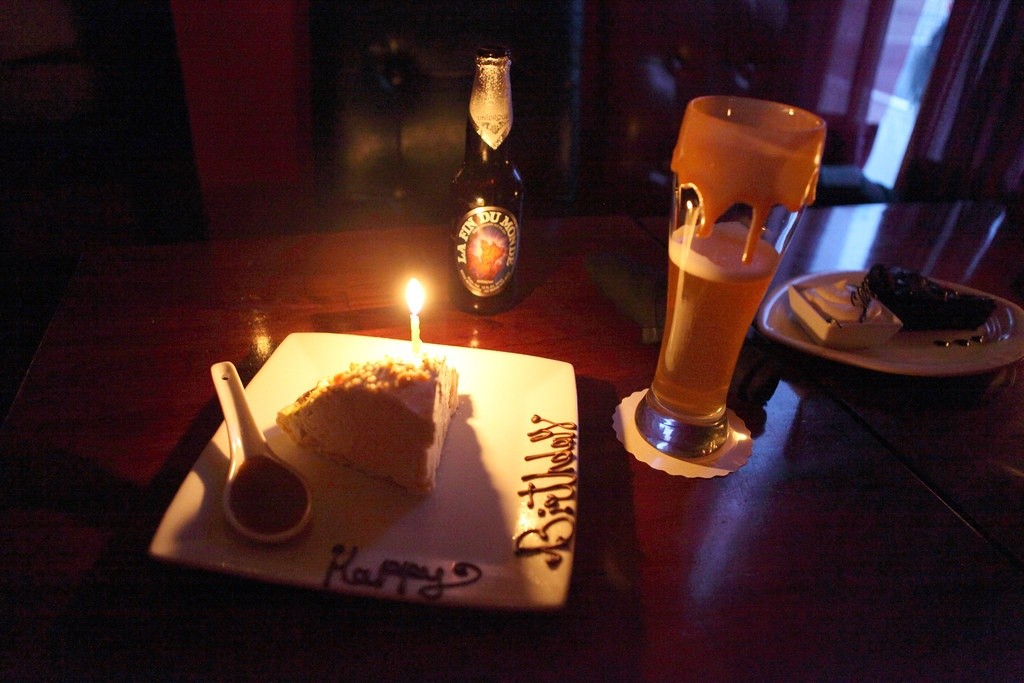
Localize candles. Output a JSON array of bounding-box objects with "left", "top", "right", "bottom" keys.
[{"left": 405, "top": 275, "right": 424, "bottom": 358}]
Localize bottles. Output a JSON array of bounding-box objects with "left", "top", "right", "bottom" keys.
[{"left": 446, "top": 46, "right": 524, "bottom": 317}]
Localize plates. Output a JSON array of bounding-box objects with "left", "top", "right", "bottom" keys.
[
  {"left": 148, "top": 331, "right": 580, "bottom": 610},
  {"left": 759, "top": 268, "right": 1024, "bottom": 377}
]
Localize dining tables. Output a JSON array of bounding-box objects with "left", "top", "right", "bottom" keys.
[{"left": 0, "top": 196, "right": 1024, "bottom": 683}]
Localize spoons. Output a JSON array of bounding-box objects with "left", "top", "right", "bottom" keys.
[{"left": 210, "top": 360, "right": 314, "bottom": 543}]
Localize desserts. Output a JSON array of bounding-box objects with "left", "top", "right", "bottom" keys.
[
  {"left": 862, "top": 263, "right": 997, "bottom": 332},
  {"left": 798, "top": 278, "right": 893, "bottom": 327},
  {"left": 275, "top": 350, "right": 459, "bottom": 495}
]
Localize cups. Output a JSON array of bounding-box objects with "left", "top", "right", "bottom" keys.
[{"left": 636, "top": 95, "right": 827, "bottom": 460}]
